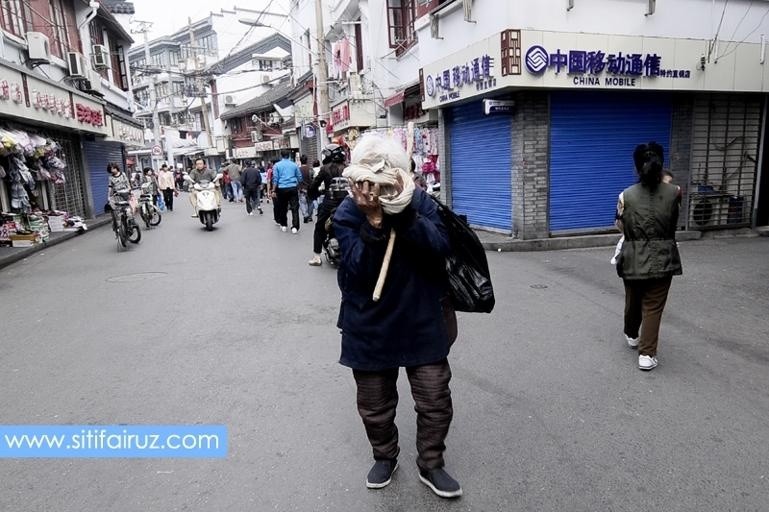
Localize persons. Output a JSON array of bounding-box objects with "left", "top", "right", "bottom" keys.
[
  {"left": 610, "top": 168, "right": 674, "bottom": 265},
  {"left": 409, "top": 157, "right": 427, "bottom": 190},
  {"left": 331, "top": 132, "right": 464, "bottom": 498},
  {"left": 616, "top": 142, "right": 683, "bottom": 371},
  {"left": 107, "top": 143, "right": 353, "bottom": 267}
]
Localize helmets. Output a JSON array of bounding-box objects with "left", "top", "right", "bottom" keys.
[{"left": 319, "top": 144, "right": 345, "bottom": 163}]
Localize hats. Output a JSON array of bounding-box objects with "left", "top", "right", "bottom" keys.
[{"left": 245, "top": 160, "right": 256, "bottom": 167}]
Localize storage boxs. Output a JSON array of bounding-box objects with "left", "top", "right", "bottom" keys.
[{"left": 11, "top": 232, "right": 33, "bottom": 247}]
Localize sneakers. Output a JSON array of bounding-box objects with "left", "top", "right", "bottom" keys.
[
  {"left": 638, "top": 353, "right": 658, "bottom": 371},
  {"left": 10, "top": 183, "right": 31, "bottom": 208},
  {"left": 192, "top": 214, "right": 198, "bottom": 217},
  {"left": 291, "top": 227, "right": 299, "bottom": 234},
  {"left": 624, "top": 333, "right": 640, "bottom": 348},
  {"left": 309, "top": 257, "right": 322, "bottom": 266},
  {"left": 303, "top": 216, "right": 312, "bottom": 223},
  {"left": 229, "top": 196, "right": 234, "bottom": 202},
  {"left": 418, "top": 469, "right": 462, "bottom": 498},
  {"left": 257, "top": 206, "right": 263, "bottom": 214},
  {"left": 280, "top": 225, "right": 287, "bottom": 231},
  {"left": 366, "top": 459, "right": 399, "bottom": 488},
  {"left": 13, "top": 158, "right": 34, "bottom": 183}
]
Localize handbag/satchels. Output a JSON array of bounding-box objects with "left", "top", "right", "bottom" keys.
[{"left": 105, "top": 202, "right": 113, "bottom": 214}]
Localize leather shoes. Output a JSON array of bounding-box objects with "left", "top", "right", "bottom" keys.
[{"left": 16, "top": 228, "right": 32, "bottom": 234}]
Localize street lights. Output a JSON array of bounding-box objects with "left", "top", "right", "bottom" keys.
[{"left": 239, "top": 18, "right": 331, "bottom": 160}]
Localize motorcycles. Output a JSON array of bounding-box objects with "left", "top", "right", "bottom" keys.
[
  {"left": 132, "top": 181, "right": 162, "bottom": 229},
  {"left": 109, "top": 187, "right": 140, "bottom": 252},
  {"left": 301, "top": 186, "right": 341, "bottom": 269},
  {"left": 183, "top": 173, "right": 223, "bottom": 230}
]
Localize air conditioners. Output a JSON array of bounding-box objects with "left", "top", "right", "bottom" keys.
[
  {"left": 224, "top": 93, "right": 237, "bottom": 105},
  {"left": 262, "top": 74, "right": 270, "bottom": 83},
  {"left": 85, "top": 70, "right": 101, "bottom": 91},
  {"left": 348, "top": 75, "right": 361, "bottom": 102},
  {"left": 689, "top": 194, "right": 729, "bottom": 226},
  {"left": 92, "top": 44, "right": 110, "bottom": 69},
  {"left": 251, "top": 131, "right": 257, "bottom": 142},
  {"left": 67, "top": 52, "right": 87, "bottom": 79},
  {"left": 28, "top": 32, "right": 51, "bottom": 63}
]
